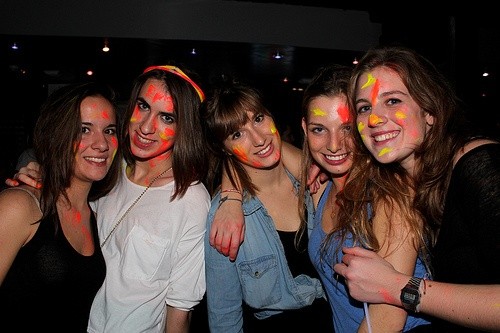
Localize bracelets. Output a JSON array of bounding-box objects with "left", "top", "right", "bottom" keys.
[
  {"left": 221, "top": 189, "right": 243, "bottom": 195},
  {"left": 219, "top": 198, "right": 243, "bottom": 207}
]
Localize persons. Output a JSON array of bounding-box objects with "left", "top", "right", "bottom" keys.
[
  {"left": 209, "top": 65, "right": 436, "bottom": 333},
  {"left": 334, "top": 47, "right": 500, "bottom": 333},
  {"left": 0, "top": 82, "right": 122, "bottom": 333},
  {"left": 5, "top": 62, "right": 212, "bottom": 333},
  {"left": 204, "top": 76, "right": 335, "bottom": 333}
]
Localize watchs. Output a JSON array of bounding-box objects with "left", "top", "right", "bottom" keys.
[{"left": 400, "top": 276, "right": 422, "bottom": 313}]
